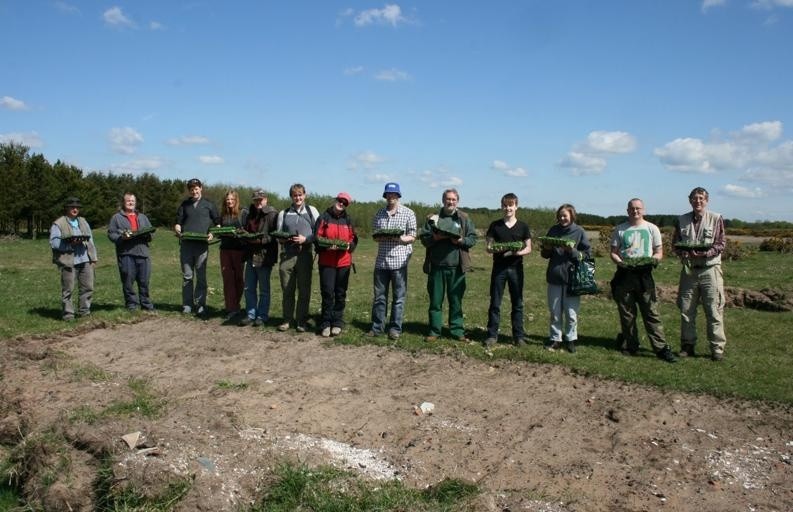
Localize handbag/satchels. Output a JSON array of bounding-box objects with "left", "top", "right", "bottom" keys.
[{"left": 567, "top": 252, "right": 596, "bottom": 295}]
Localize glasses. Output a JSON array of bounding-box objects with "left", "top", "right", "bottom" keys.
[
  {"left": 691, "top": 197, "right": 709, "bottom": 202},
  {"left": 629, "top": 207, "right": 644, "bottom": 212},
  {"left": 337, "top": 199, "right": 348, "bottom": 208}
]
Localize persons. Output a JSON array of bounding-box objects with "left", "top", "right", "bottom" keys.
[
  {"left": 485, "top": 193, "right": 532, "bottom": 345},
  {"left": 540, "top": 204, "right": 592, "bottom": 354},
  {"left": 277, "top": 184, "right": 320, "bottom": 332},
  {"left": 174, "top": 179, "right": 220, "bottom": 314},
  {"left": 108, "top": 191, "right": 156, "bottom": 311},
  {"left": 50, "top": 197, "right": 97, "bottom": 321},
  {"left": 240, "top": 190, "right": 279, "bottom": 326},
  {"left": 672, "top": 188, "right": 726, "bottom": 362},
  {"left": 364, "top": 183, "right": 417, "bottom": 340},
  {"left": 611, "top": 199, "right": 674, "bottom": 360},
  {"left": 419, "top": 189, "right": 478, "bottom": 343},
  {"left": 217, "top": 189, "right": 249, "bottom": 318},
  {"left": 314, "top": 192, "right": 358, "bottom": 337}
]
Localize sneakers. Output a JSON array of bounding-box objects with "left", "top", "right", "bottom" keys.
[
  {"left": 331, "top": 327, "right": 342, "bottom": 336},
  {"left": 254, "top": 316, "right": 266, "bottom": 326},
  {"left": 483, "top": 336, "right": 501, "bottom": 346},
  {"left": 323, "top": 326, "right": 331, "bottom": 337},
  {"left": 388, "top": 331, "right": 401, "bottom": 340},
  {"left": 238, "top": 314, "right": 254, "bottom": 326},
  {"left": 513, "top": 336, "right": 529, "bottom": 348},
  {"left": 363, "top": 329, "right": 380, "bottom": 337},
  {"left": 658, "top": 345, "right": 679, "bottom": 363},
  {"left": 544, "top": 341, "right": 563, "bottom": 350},
  {"left": 142, "top": 303, "right": 155, "bottom": 310},
  {"left": 623, "top": 345, "right": 640, "bottom": 355},
  {"left": 566, "top": 341, "right": 578, "bottom": 353},
  {"left": 713, "top": 349, "right": 725, "bottom": 362},
  {"left": 451, "top": 334, "right": 470, "bottom": 342},
  {"left": 182, "top": 306, "right": 193, "bottom": 314},
  {"left": 278, "top": 319, "right": 295, "bottom": 331},
  {"left": 425, "top": 336, "right": 441, "bottom": 343},
  {"left": 128, "top": 304, "right": 138, "bottom": 312},
  {"left": 197, "top": 306, "right": 206, "bottom": 314},
  {"left": 679, "top": 344, "right": 696, "bottom": 357},
  {"left": 297, "top": 322, "right": 307, "bottom": 332}
]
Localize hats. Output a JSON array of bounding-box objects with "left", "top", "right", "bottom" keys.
[
  {"left": 63, "top": 197, "right": 82, "bottom": 207},
  {"left": 383, "top": 183, "right": 402, "bottom": 198},
  {"left": 188, "top": 179, "right": 201, "bottom": 188},
  {"left": 336, "top": 192, "right": 351, "bottom": 206},
  {"left": 252, "top": 190, "right": 266, "bottom": 200}
]
